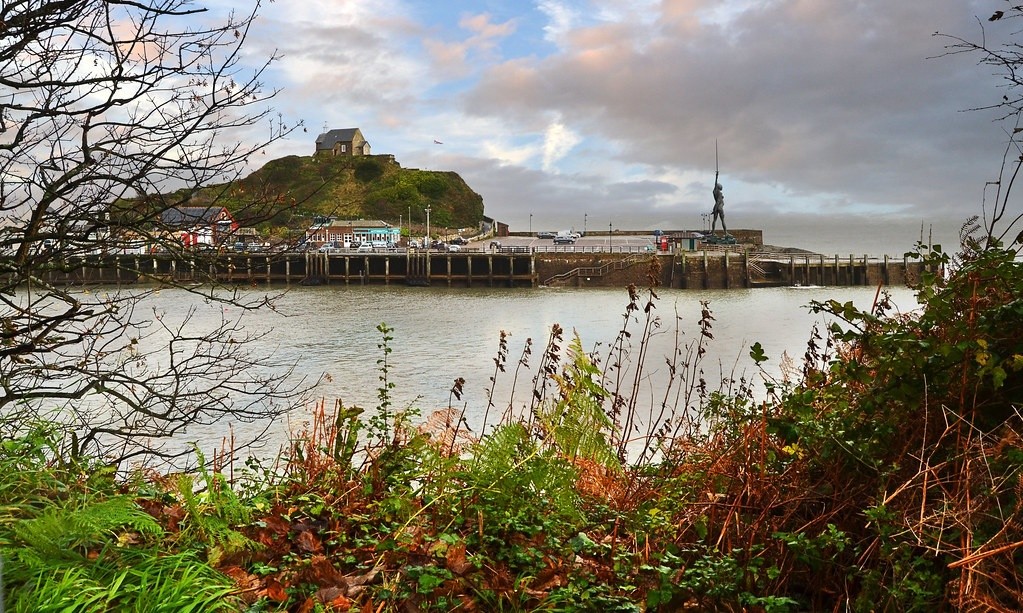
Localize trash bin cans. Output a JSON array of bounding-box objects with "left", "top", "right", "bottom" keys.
[
  {"left": 492, "top": 246, "right": 497, "bottom": 254},
  {"left": 410, "top": 248, "right": 415, "bottom": 257},
  {"left": 668, "top": 243, "right": 674, "bottom": 254}
]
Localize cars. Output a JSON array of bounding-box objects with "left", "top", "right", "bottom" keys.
[
  {"left": 449, "top": 244, "right": 462, "bottom": 252},
  {"left": 318, "top": 243, "right": 334, "bottom": 253},
  {"left": 450, "top": 237, "right": 468, "bottom": 245},
  {"left": 409, "top": 237, "right": 445, "bottom": 251},
  {"left": 357, "top": 242, "right": 372, "bottom": 253},
  {"left": 537, "top": 230, "right": 581, "bottom": 244},
  {"left": 489, "top": 241, "right": 502, "bottom": 249}
]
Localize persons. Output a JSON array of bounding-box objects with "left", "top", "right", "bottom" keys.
[{"left": 709, "top": 174, "right": 733, "bottom": 236}]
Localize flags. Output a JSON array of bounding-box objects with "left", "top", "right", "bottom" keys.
[{"left": 433, "top": 139, "right": 443, "bottom": 144}]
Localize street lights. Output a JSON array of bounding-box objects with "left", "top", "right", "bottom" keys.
[
  {"left": 608, "top": 221, "right": 613, "bottom": 253},
  {"left": 445, "top": 226, "right": 448, "bottom": 244},
  {"left": 408, "top": 206, "right": 411, "bottom": 248},
  {"left": 399, "top": 214, "right": 403, "bottom": 231},
  {"left": 584, "top": 212, "right": 588, "bottom": 236},
  {"left": 424, "top": 204, "right": 432, "bottom": 250},
  {"left": 529, "top": 213, "right": 533, "bottom": 236}
]
